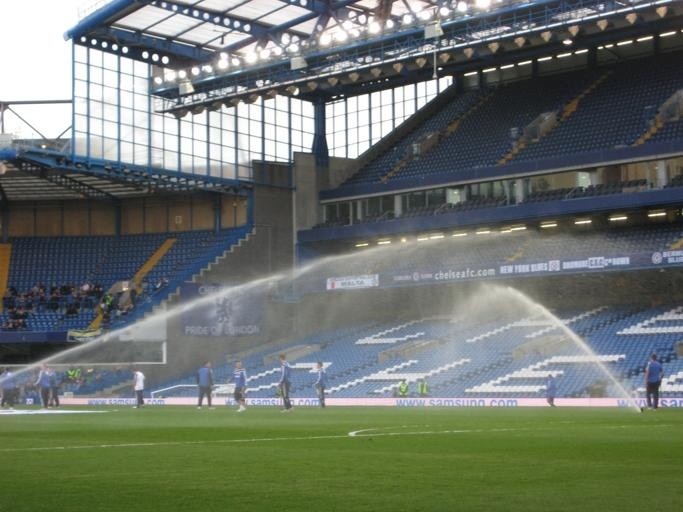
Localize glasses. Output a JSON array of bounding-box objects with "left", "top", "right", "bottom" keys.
[{"left": 423, "top": 8, "right": 445, "bottom": 39}]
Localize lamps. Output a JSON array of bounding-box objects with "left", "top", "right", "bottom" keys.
[{"left": 423, "top": 8, "right": 445, "bottom": 39}]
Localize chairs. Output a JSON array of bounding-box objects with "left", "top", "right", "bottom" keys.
[
  {"left": 196, "top": 406, "right": 216, "bottom": 410},
  {"left": 0, "top": 225, "right": 249, "bottom": 351},
  {"left": 2, "top": 312, "right": 682, "bottom": 398},
  {"left": 236, "top": 405, "right": 246, "bottom": 412},
  {"left": 133, "top": 404, "right": 145, "bottom": 408},
  {"left": 296, "top": 65, "right": 683, "bottom": 292},
  {"left": 280, "top": 407, "right": 294, "bottom": 413}
]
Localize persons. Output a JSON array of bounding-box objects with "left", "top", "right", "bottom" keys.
[
  {"left": 417, "top": 379, "right": 429, "bottom": 397},
  {"left": 0, "top": 277, "right": 169, "bottom": 409},
  {"left": 278, "top": 355, "right": 293, "bottom": 413},
  {"left": 313, "top": 362, "right": 326, "bottom": 408},
  {"left": 399, "top": 380, "right": 408, "bottom": 397},
  {"left": 544, "top": 374, "right": 556, "bottom": 406},
  {"left": 196, "top": 361, "right": 214, "bottom": 409},
  {"left": 228, "top": 361, "right": 247, "bottom": 413},
  {"left": 644, "top": 354, "right": 663, "bottom": 411}
]
